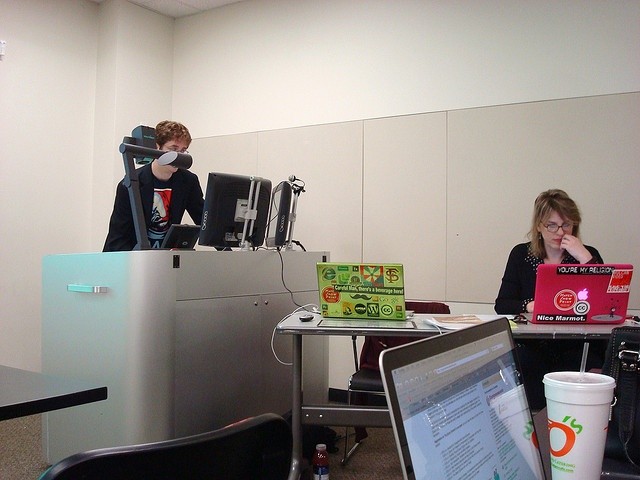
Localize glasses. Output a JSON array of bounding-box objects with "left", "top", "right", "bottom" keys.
[
  {"left": 541, "top": 220, "right": 572, "bottom": 233},
  {"left": 164, "top": 145, "right": 189, "bottom": 154}
]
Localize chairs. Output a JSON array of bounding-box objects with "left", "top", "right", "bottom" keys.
[{"left": 341, "top": 303, "right": 451, "bottom": 466}]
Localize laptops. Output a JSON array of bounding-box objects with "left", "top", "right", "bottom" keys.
[
  {"left": 315, "top": 261, "right": 414, "bottom": 324},
  {"left": 520, "top": 262, "right": 634, "bottom": 329}
]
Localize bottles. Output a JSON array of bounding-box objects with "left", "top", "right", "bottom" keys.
[{"left": 313, "top": 442, "right": 329, "bottom": 480}]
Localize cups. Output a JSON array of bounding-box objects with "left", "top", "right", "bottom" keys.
[{"left": 543, "top": 371, "right": 618, "bottom": 480}]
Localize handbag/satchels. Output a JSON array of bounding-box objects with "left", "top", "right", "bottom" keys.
[{"left": 600, "top": 325, "right": 640, "bottom": 467}]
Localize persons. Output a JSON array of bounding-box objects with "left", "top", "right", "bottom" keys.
[
  {"left": 494, "top": 189, "right": 610, "bottom": 411},
  {"left": 103, "top": 121, "right": 233, "bottom": 251}
]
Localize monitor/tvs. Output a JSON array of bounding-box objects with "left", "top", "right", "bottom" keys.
[
  {"left": 198, "top": 171, "right": 273, "bottom": 253},
  {"left": 264, "top": 177, "right": 307, "bottom": 252}
]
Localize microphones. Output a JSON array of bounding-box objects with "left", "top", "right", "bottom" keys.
[{"left": 288, "top": 173, "right": 300, "bottom": 190}]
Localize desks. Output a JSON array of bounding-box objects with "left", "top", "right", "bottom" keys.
[
  {"left": 1, "top": 366, "right": 108, "bottom": 440},
  {"left": 277, "top": 308, "right": 640, "bottom": 476}
]
